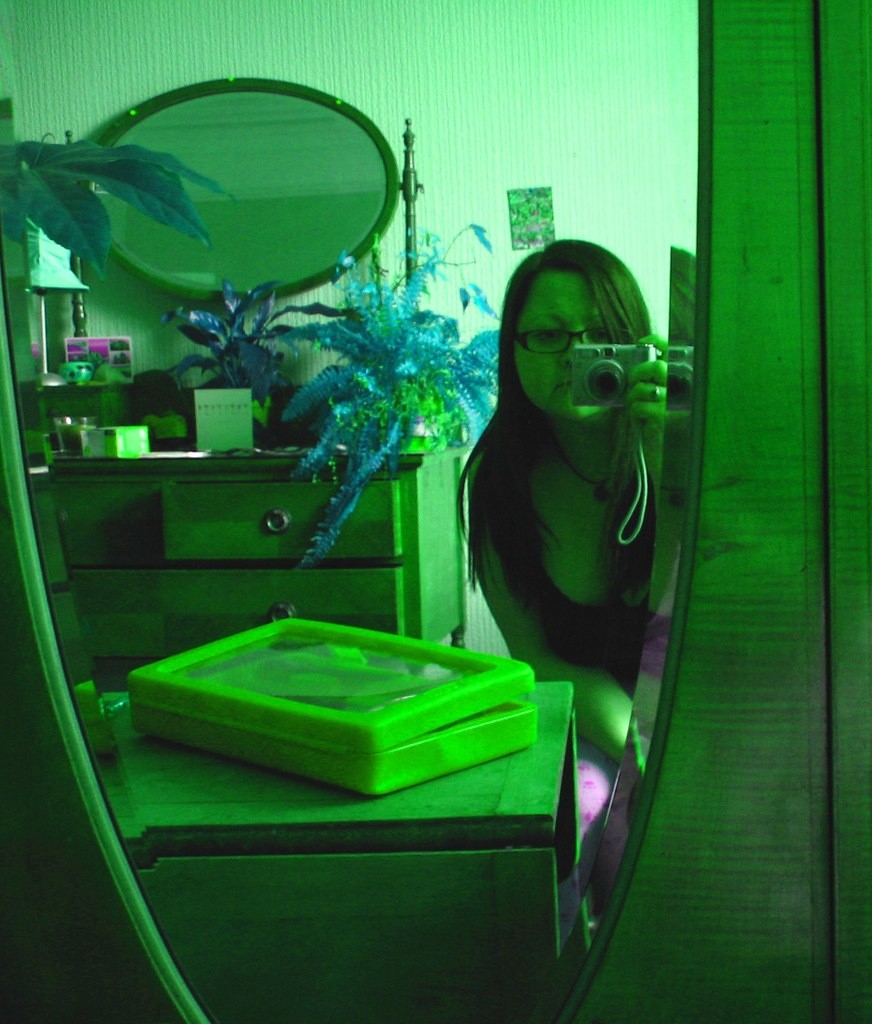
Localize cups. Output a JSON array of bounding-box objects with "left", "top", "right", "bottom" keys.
[
  {"left": 54, "top": 415, "right": 96, "bottom": 456},
  {"left": 57, "top": 362, "right": 94, "bottom": 387},
  {"left": 79, "top": 428, "right": 105, "bottom": 456}
]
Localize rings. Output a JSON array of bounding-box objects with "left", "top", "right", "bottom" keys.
[{"left": 654, "top": 383, "right": 662, "bottom": 402}]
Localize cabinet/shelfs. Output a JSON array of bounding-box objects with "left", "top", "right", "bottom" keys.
[{"left": 53, "top": 440, "right": 467, "bottom": 653}]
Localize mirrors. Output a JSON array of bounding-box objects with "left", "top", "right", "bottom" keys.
[
  {"left": 86, "top": 77, "right": 397, "bottom": 301},
  {"left": 0, "top": 0, "right": 708, "bottom": 1024}
]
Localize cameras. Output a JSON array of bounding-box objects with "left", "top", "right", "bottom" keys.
[{"left": 571, "top": 343, "right": 656, "bottom": 405}]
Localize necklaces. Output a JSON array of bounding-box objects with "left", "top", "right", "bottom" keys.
[{"left": 553, "top": 432, "right": 618, "bottom": 505}]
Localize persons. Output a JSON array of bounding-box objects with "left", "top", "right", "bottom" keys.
[{"left": 458, "top": 243, "right": 671, "bottom": 770}]
